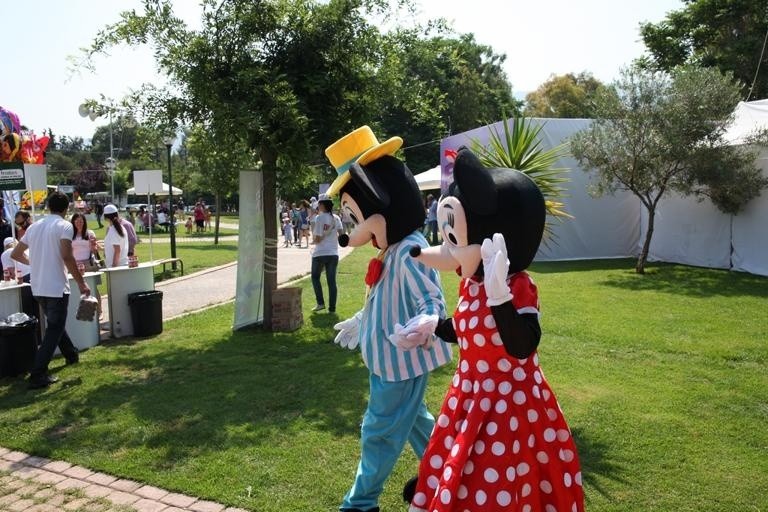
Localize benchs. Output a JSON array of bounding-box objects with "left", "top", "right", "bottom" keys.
[{"left": 159, "top": 257, "right": 184, "bottom": 276}]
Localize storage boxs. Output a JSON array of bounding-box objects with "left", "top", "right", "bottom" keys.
[
  {"left": 271, "top": 287, "right": 304, "bottom": 316},
  {"left": 272, "top": 312, "right": 304, "bottom": 333}
]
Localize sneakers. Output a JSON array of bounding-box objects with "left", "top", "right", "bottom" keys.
[
  {"left": 311, "top": 304, "right": 337, "bottom": 316},
  {"left": 284, "top": 238, "right": 311, "bottom": 249}
]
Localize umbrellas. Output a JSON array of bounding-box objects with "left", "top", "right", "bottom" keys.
[
  {"left": 126, "top": 182, "right": 183, "bottom": 217},
  {"left": 412, "top": 165, "right": 442, "bottom": 190}
]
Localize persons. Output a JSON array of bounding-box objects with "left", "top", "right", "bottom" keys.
[
  {"left": 134, "top": 200, "right": 209, "bottom": 234},
  {"left": 70, "top": 198, "right": 136, "bottom": 331},
  {"left": 11, "top": 193, "right": 92, "bottom": 384},
  {"left": 1, "top": 209, "right": 33, "bottom": 284},
  {"left": 310, "top": 194, "right": 346, "bottom": 314},
  {"left": 278, "top": 192, "right": 437, "bottom": 249}
]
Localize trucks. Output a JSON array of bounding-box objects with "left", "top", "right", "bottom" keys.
[{"left": 35, "top": 185, "right": 74, "bottom": 211}]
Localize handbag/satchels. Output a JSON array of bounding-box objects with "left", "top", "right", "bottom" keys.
[
  {"left": 301, "top": 224, "right": 310, "bottom": 229},
  {"left": 90, "top": 253, "right": 106, "bottom": 273}
]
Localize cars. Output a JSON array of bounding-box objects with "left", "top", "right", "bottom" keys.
[{"left": 86, "top": 191, "right": 215, "bottom": 214}]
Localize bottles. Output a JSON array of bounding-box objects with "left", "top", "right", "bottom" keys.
[
  {"left": 127, "top": 256, "right": 139, "bottom": 268},
  {"left": 3, "top": 269, "right": 11, "bottom": 285},
  {"left": 76, "top": 262, "right": 85, "bottom": 275},
  {"left": 16, "top": 270, "right": 23, "bottom": 284}
]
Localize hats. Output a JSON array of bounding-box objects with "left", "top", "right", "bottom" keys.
[
  {"left": 102, "top": 204, "right": 118, "bottom": 215},
  {"left": 281, "top": 211, "right": 291, "bottom": 223},
  {"left": 317, "top": 193, "right": 333, "bottom": 203}
]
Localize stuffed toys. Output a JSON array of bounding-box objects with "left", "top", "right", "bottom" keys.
[
  {"left": 399, "top": 145, "right": 583, "bottom": 511},
  {"left": 324, "top": 125, "right": 454, "bottom": 512}
]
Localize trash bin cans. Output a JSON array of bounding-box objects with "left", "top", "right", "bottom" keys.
[
  {"left": 0, "top": 312, "right": 38, "bottom": 376},
  {"left": 128, "top": 290, "right": 163, "bottom": 337}
]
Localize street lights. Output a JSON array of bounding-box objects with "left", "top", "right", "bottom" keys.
[{"left": 159, "top": 117, "right": 178, "bottom": 271}]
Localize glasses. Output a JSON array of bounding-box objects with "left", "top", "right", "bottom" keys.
[
  {"left": 74, "top": 219, "right": 85, "bottom": 223},
  {"left": 14, "top": 218, "right": 27, "bottom": 225}
]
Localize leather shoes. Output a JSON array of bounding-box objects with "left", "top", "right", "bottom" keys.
[
  {"left": 66, "top": 348, "right": 79, "bottom": 362},
  {"left": 31, "top": 372, "right": 59, "bottom": 383}
]
